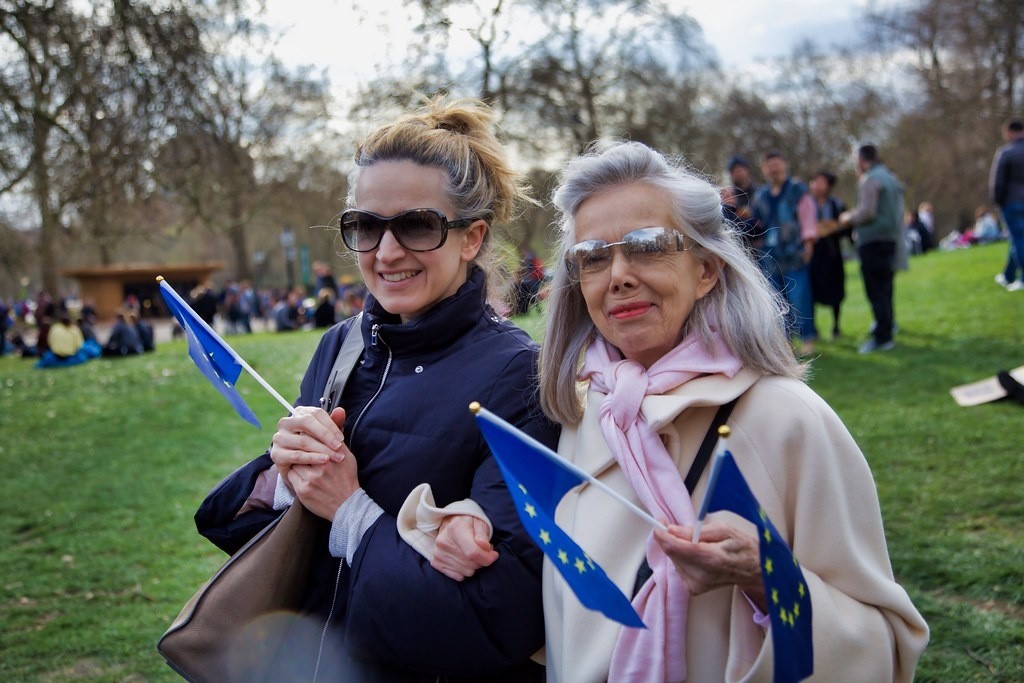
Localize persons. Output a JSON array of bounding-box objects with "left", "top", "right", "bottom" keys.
[
  {"left": 719, "top": 144, "right": 909, "bottom": 351},
  {"left": 898, "top": 196, "right": 999, "bottom": 254},
  {"left": 398, "top": 140, "right": 931, "bottom": 683},
  {"left": 986, "top": 119, "right": 1023, "bottom": 292},
  {"left": 0, "top": 261, "right": 367, "bottom": 370},
  {"left": 510, "top": 244, "right": 545, "bottom": 318},
  {"left": 192, "top": 100, "right": 563, "bottom": 683}
]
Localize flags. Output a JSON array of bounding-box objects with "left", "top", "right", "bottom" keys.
[
  {"left": 158, "top": 280, "right": 263, "bottom": 431},
  {"left": 476, "top": 408, "right": 647, "bottom": 631},
  {"left": 700, "top": 452, "right": 816, "bottom": 683}
]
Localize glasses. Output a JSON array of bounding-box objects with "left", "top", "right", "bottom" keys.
[
  {"left": 341, "top": 209, "right": 492, "bottom": 252},
  {"left": 564, "top": 227, "right": 705, "bottom": 281}
]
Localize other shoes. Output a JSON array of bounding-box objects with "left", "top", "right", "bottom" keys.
[
  {"left": 995, "top": 272, "right": 1009, "bottom": 288},
  {"left": 857, "top": 338, "right": 895, "bottom": 354},
  {"left": 1006, "top": 278, "right": 1024, "bottom": 292}
]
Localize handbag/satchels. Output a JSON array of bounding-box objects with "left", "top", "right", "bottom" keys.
[{"left": 156, "top": 311, "right": 365, "bottom": 683}]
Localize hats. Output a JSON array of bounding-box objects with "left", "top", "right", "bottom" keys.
[{"left": 727, "top": 156, "right": 747, "bottom": 173}]
